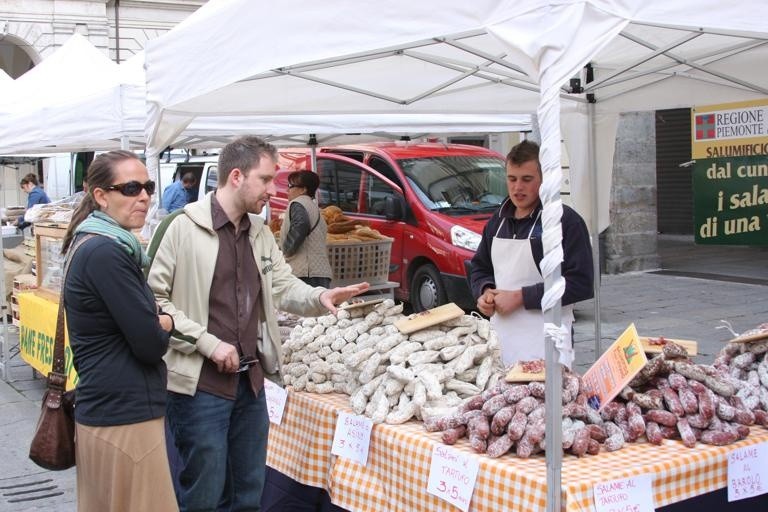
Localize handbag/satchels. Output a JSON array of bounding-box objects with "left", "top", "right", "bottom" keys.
[{"left": 29, "top": 388, "right": 76, "bottom": 471}]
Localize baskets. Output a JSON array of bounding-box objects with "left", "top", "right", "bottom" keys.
[{"left": 327, "top": 238, "right": 395, "bottom": 285}]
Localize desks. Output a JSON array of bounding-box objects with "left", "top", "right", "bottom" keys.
[
  {"left": 17, "top": 292, "right": 78, "bottom": 392},
  {"left": 351, "top": 280, "right": 401, "bottom": 306},
  {"left": 259, "top": 365, "right": 766, "bottom": 511}
]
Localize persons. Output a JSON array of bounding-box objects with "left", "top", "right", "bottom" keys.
[
  {"left": 278, "top": 170, "right": 332, "bottom": 288},
  {"left": 60, "top": 148, "right": 180, "bottom": 512},
  {"left": 146, "top": 139, "right": 370, "bottom": 512},
  {"left": 11, "top": 172, "right": 52, "bottom": 230},
  {"left": 157, "top": 171, "right": 198, "bottom": 212},
  {"left": 468, "top": 138, "right": 599, "bottom": 368}
]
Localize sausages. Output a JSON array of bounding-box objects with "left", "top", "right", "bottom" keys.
[
  {"left": 425, "top": 323, "right": 768, "bottom": 458},
  {"left": 279, "top": 298, "right": 504, "bottom": 426}
]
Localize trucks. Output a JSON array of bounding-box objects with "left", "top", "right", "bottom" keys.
[
  {"left": 42, "top": 149, "right": 267, "bottom": 223},
  {"left": 266, "top": 136, "right": 510, "bottom": 311}
]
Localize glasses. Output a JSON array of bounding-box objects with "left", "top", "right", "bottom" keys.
[
  {"left": 236, "top": 354, "right": 259, "bottom": 375},
  {"left": 94, "top": 181, "right": 156, "bottom": 197}
]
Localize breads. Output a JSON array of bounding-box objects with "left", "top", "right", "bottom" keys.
[{"left": 322, "top": 206, "right": 384, "bottom": 242}]
